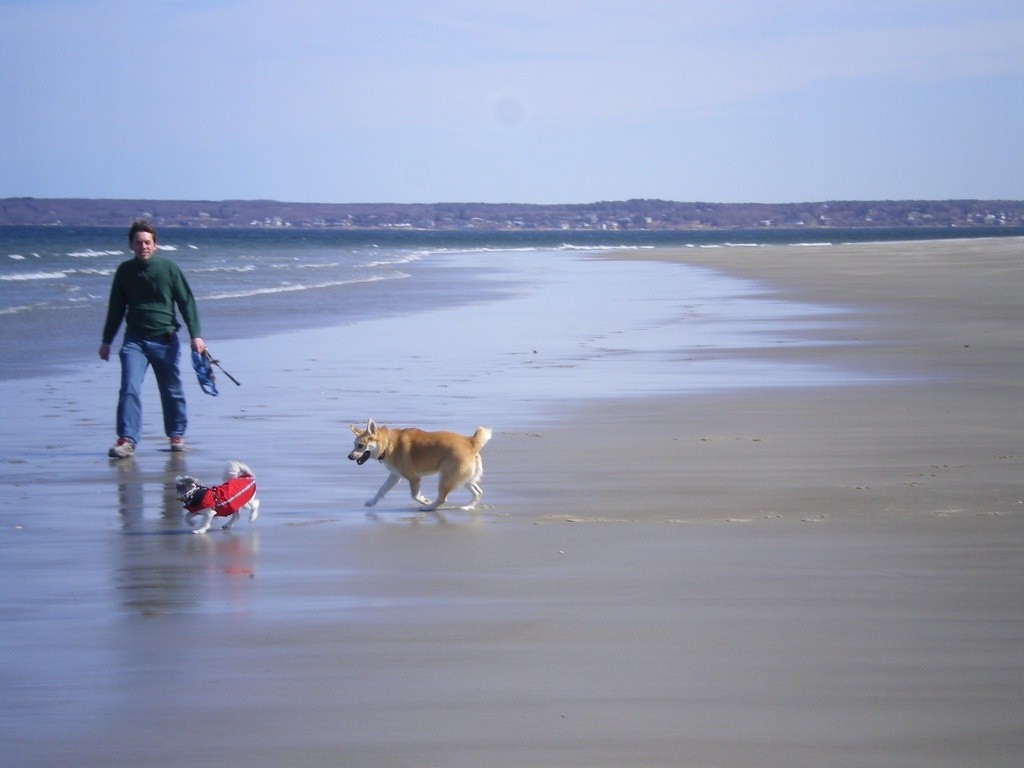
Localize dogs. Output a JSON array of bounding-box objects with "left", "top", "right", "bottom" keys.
[
  {"left": 347, "top": 417, "right": 493, "bottom": 511},
  {"left": 174, "top": 459, "right": 260, "bottom": 535}
]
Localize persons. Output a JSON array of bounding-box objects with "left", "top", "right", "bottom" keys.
[{"left": 98, "top": 221, "right": 205, "bottom": 458}]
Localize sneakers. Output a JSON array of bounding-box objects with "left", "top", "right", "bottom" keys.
[
  {"left": 170, "top": 435, "right": 184, "bottom": 450},
  {"left": 109, "top": 437, "right": 134, "bottom": 457}
]
